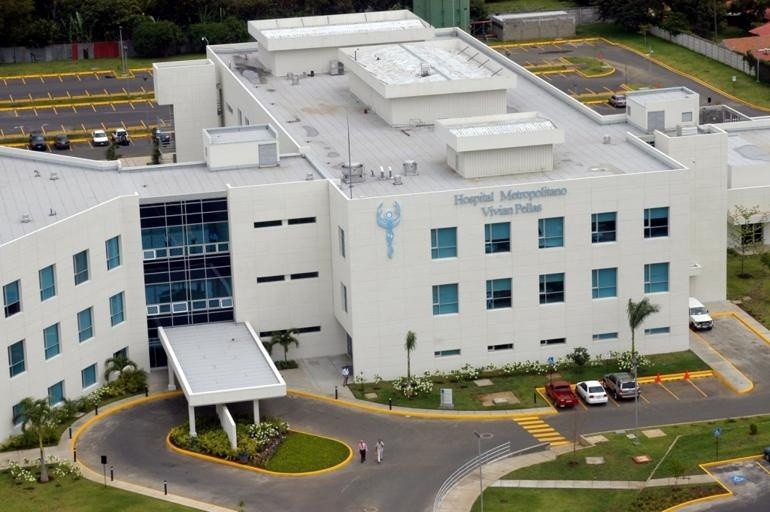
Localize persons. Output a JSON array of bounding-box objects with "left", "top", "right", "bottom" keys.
[
  {"left": 376, "top": 438, "right": 387, "bottom": 464},
  {"left": 358, "top": 439, "right": 369, "bottom": 466},
  {"left": 341, "top": 366, "right": 350, "bottom": 388}
]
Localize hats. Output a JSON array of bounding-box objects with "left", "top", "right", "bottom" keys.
[
  {"left": 30, "top": 134, "right": 70, "bottom": 151},
  {"left": 608, "top": 95, "right": 626, "bottom": 107},
  {"left": 92, "top": 128, "right": 128, "bottom": 146},
  {"left": 152, "top": 127, "right": 170, "bottom": 145},
  {"left": 545, "top": 372, "right": 642, "bottom": 409}
]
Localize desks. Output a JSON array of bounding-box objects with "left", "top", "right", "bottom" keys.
[
  {"left": 631, "top": 356, "right": 638, "bottom": 444},
  {"left": 473, "top": 431, "right": 494, "bottom": 512}
]
